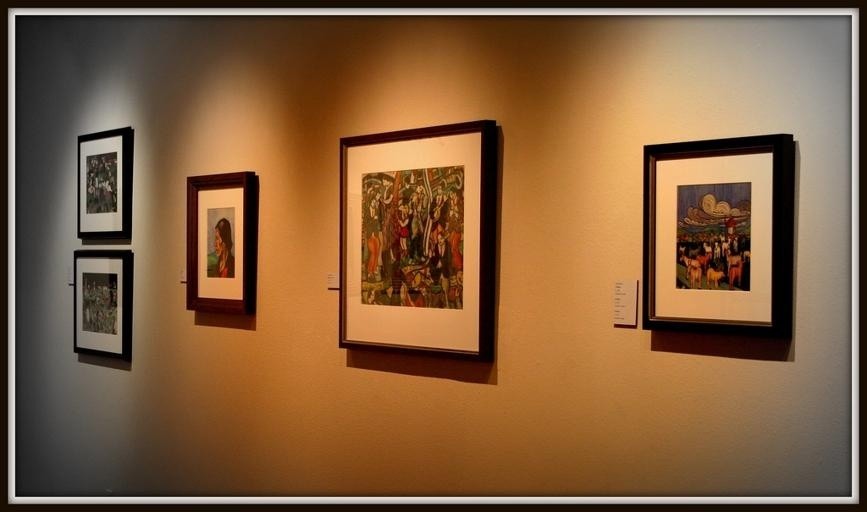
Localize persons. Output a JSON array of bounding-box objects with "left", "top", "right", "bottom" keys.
[
  {"left": 213, "top": 218, "right": 235, "bottom": 278},
  {"left": 362, "top": 173, "right": 466, "bottom": 310}
]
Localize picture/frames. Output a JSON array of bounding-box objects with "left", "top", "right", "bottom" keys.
[{"left": 72, "top": 125, "right": 136, "bottom": 362}]
